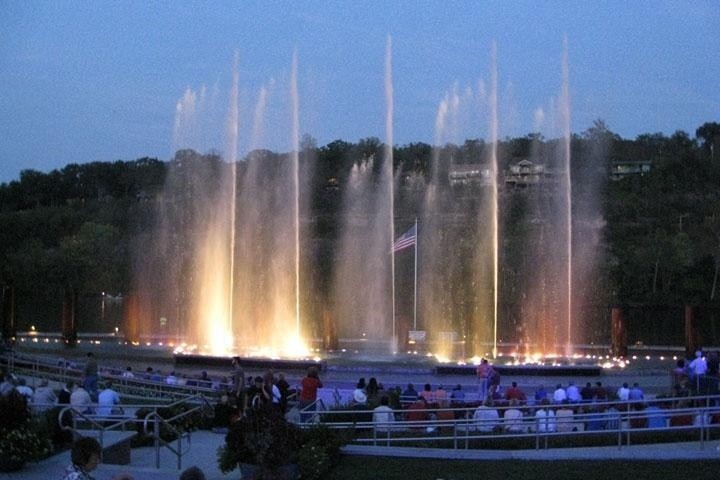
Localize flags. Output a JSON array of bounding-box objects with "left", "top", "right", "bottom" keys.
[{"left": 387, "top": 223, "right": 417, "bottom": 255}]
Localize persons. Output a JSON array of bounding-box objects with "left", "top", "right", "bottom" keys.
[
  {"left": 1, "top": 349, "right": 720, "bottom": 434},
  {"left": 60, "top": 437, "right": 102, "bottom": 480},
  {"left": 179, "top": 466, "right": 205, "bottom": 480},
  {"left": 110, "top": 473, "right": 133, "bottom": 480}
]
